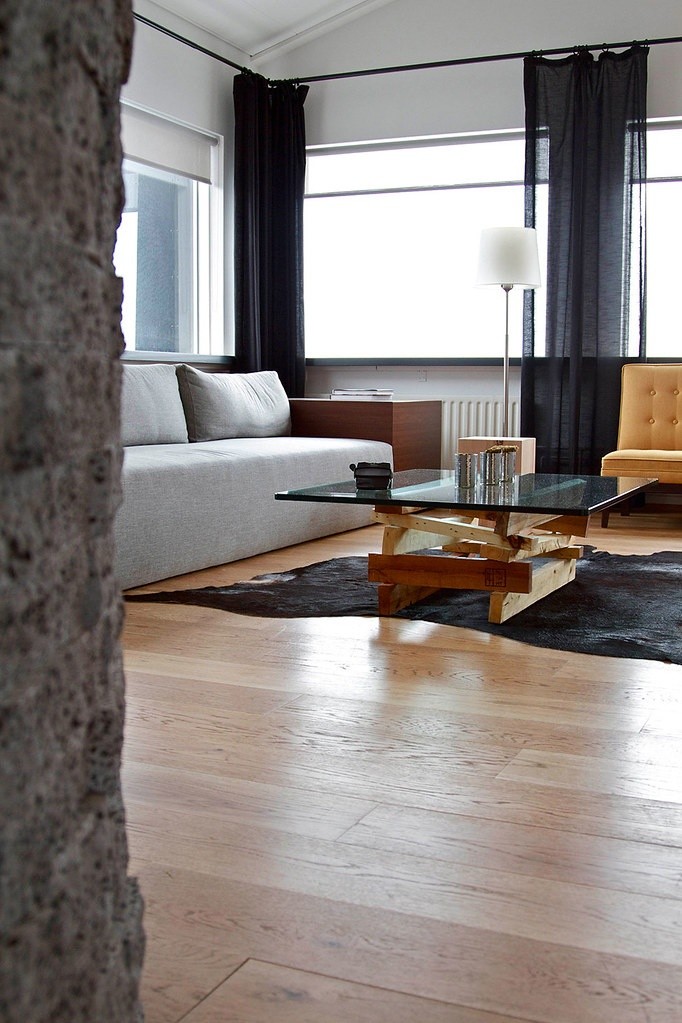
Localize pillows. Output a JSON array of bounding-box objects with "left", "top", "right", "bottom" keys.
[
  {"left": 176, "top": 361, "right": 292, "bottom": 443},
  {"left": 119, "top": 364, "right": 188, "bottom": 446}
]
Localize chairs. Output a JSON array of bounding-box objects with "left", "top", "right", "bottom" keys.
[{"left": 600, "top": 362, "right": 682, "bottom": 528}]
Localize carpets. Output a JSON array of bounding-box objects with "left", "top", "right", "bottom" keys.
[{"left": 123, "top": 544, "right": 682, "bottom": 666}]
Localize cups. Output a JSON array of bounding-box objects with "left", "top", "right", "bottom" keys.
[
  {"left": 501, "top": 452, "right": 516, "bottom": 482},
  {"left": 454, "top": 453, "right": 478, "bottom": 488},
  {"left": 480, "top": 452, "right": 502, "bottom": 485}
]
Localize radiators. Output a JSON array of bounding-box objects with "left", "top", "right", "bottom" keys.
[{"left": 441, "top": 395, "right": 521, "bottom": 469}]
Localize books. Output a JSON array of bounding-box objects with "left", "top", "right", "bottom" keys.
[
  {"left": 330, "top": 395, "right": 392, "bottom": 401},
  {"left": 331, "top": 388, "right": 395, "bottom": 395}
]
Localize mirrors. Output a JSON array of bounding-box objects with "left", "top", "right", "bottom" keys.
[{"left": 113, "top": 99, "right": 243, "bottom": 365}]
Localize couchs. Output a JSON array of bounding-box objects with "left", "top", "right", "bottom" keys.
[{"left": 114, "top": 435, "right": 395, "bottom": 593}]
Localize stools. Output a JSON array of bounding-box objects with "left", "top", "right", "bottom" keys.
[{"left": 458, "top": 437, "right": 536, "bottom": 474}]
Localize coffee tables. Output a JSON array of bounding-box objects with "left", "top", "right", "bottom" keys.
[{"left": 274, "top": 469, "right": 661, "bottom": 624}]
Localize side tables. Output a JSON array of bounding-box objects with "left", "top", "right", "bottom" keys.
[{"left": 288, "top": 398, "right": 441, "bottom": 472}]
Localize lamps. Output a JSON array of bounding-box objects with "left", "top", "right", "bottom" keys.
[{"left": 475, "top": 227, "right": 541, "bottom": 436}]
